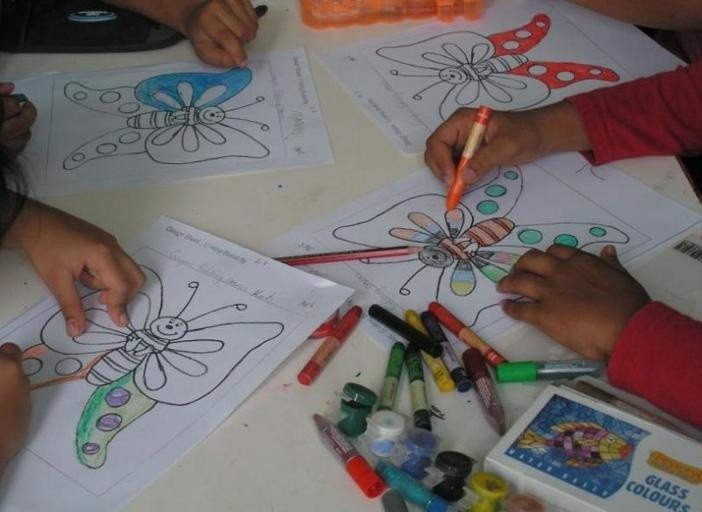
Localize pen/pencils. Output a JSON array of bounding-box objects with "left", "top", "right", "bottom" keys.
[
  {"left": 275, "top": 247, "right": 423, "bottom": 265},
  {"left": 255, "top": 5, "right": 268, "bottom": 17},
  {"left": 298, "top": 303, "right": 363, "bottom": 385},
  {"left": 374, "top": 458, "right": 446, "bottom": 511},
  {"left": 447, "top": 105, "right": 493, "bottom": 210},
  {"left": 367, "top": 303, "right": 507, "bottom": 438},
  {"left": 309, "top": 414, "right": 382, "bottom": 501},
  {"left": 496, "top": 360, "right": 608, "bottom": 383}
]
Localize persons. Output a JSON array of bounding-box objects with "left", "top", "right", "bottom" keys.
[
  {"left": 1, "top": 0, "right": 268, "bottom": 70},
  {"left": 0, "top": 185, "right": 147, "bottom": 487},
  {"left": 1, "top": 79, "right": 38, "bottom": 164},
  {"left": 562, "top": 0, "right": 700, "bottom": 31},
  {"left": 423, "top": 60, "right": 702, "bottom": 434}
]
